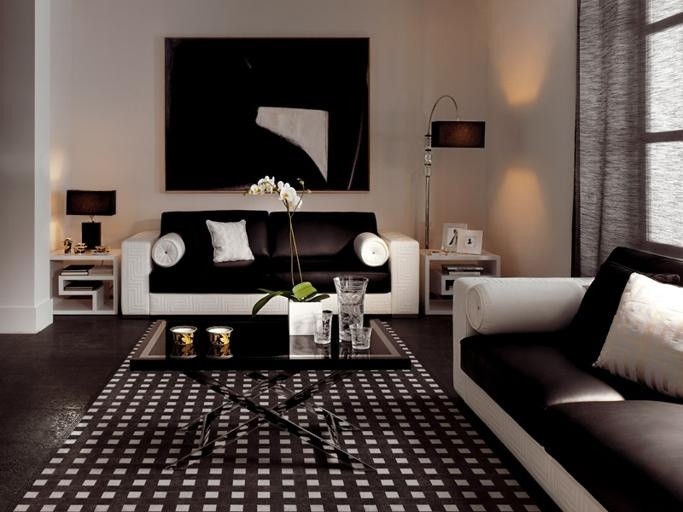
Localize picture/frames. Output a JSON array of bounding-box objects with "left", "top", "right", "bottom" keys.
[
  {"left": 440, "top": 220, "right": 469, "bottom": 253},
  {"left": 454, "top": 229, "right": 484, "bottom": 257}
]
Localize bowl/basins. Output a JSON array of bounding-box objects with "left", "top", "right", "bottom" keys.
[
  {"left": 207, "top": 346, "right": 233, "bottom": 359},
  {"left": 170, "top": 326, "right": 197, "bottom": 345},
  {"left": 171, "top": 345, "right": 198, "bottom": 359},
  {"left": 206, "top": 326, "right": 233, "bottom": 345}
]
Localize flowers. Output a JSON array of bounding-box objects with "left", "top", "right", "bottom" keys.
[{"left": 239, "top": 170, "right": 332, "bottom": 320}]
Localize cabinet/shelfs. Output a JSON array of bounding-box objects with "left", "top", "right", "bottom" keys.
[
  {"left": 415, "top": 249, "right": 501, "bottom": 317},
  {"left": 44, "top": 247, "right": 122, "bottom": 316}
]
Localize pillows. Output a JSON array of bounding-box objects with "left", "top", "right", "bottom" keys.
[
  {"left": 199, "top": 214, "right": 257, "bottom": 265},
  {"left": 552, "top": 255, "right": 681, "bottom": 401},
  {"left": 462, "top": 272, "right": 587, "bottom": 338},
  {"left": 346, "top": 230, "right": 391, "bottom": 268},
  {"left": 584, "top": 264, "right": 683, "bottom": 395},
  {"left": 150, "top": 227, "right": 188, "bottom": 271}
]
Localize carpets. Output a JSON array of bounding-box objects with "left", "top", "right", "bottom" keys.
[{"left": 4, "top": 314, "right": 560, "bottom": 511}]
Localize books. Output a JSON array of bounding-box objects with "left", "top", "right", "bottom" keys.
[
  {"left": 446, "top": 270, "right": 482, "bottom": 277},
  {"left": 441, "top": 262, "right": 484, "bottom": 271},
  {"left": 87, "top": 265, "right": 114, "bottom": 276},
  {"left": 63, "top": 282, "right": 102, "bottom": 291},
  {"left": 59, "top": 265, "right": 90, "bottom": 276}
]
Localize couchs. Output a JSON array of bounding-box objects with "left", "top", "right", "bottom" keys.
[
  {"left": 114, "top": 206, "right": 424, "bottom": 323},
  {"left": 434, "top": 240, "right": 681, "bottom": 512}
]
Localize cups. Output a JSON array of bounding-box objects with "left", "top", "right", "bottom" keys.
[
  {"left": 348, "top": 323, "right": 371, "bottom": 349},
  {"left": 314, "top": 345, "right": 332, "bottom": 360},
  {"left": 63, "top": 238, "right": 109, "bottom": 255},
  {"left": 312, "top": 313, "right": 333, "bottom": 345},
  {"left": 338, "top": 341, "right": 371, "bottom": 358},
  {"left": 332, "top": 274, "right": 369, "bottom": 342}
]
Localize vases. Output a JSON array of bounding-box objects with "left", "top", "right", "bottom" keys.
[
  {"left": 281, "top": 295, "right": 327, "bottom": 338},
  {"left": 286, "top": 335, "right": 326, "bottom": 362}
]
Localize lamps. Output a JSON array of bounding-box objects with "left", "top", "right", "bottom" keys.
[
  {"left": 420, "top": 92, "right": 489, "bottom": 253},
  {"left": 62, "top": 184, "right": 119, "bottom": 252}
]
